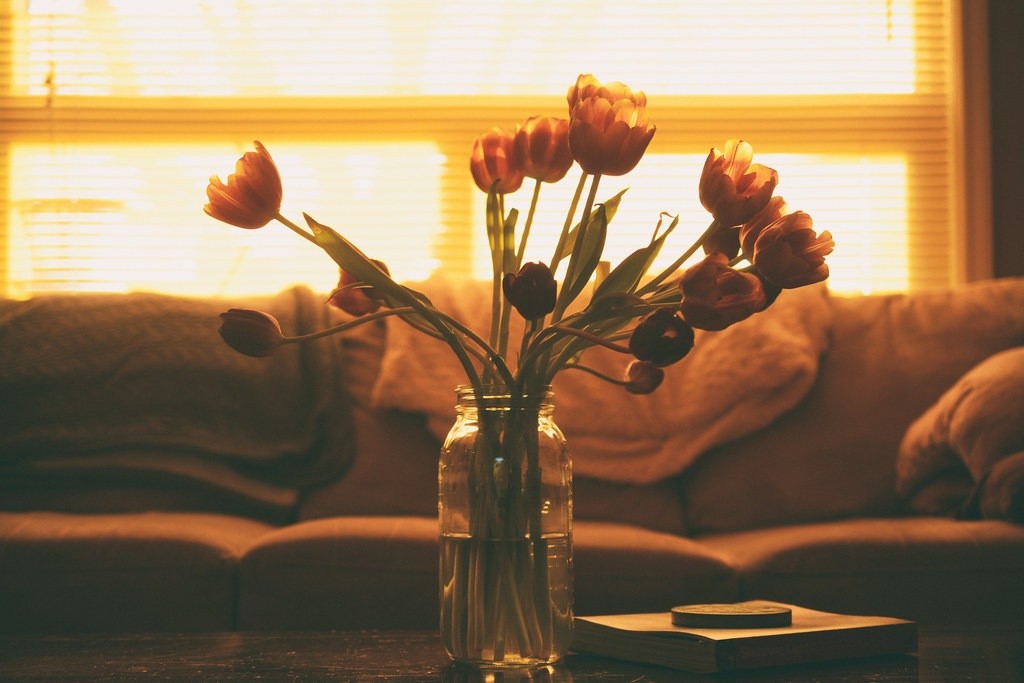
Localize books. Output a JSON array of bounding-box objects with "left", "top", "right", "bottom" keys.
[{"left": 563, "top": 596, "right": 922, "bottom": 672}]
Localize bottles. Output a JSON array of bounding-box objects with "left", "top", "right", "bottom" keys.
[{"left": 437, "top": 385, "right": 576, "bottom": 664}]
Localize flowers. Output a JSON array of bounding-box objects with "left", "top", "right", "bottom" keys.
[{"left": 205, "top": 74, "right": 835, "bottom": 661}]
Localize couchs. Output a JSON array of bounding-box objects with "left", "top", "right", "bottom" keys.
[{"left": 0, "top": 280, "right": 1024, "bottom": 628}]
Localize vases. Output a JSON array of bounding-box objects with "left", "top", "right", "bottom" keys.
[{"left": 438, "top": 383, "right": 572, "bottom": 667}]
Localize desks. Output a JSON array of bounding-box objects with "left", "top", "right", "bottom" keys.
[{"left": 0, "top": 627, "right": 1024, "bottom": 683}]
